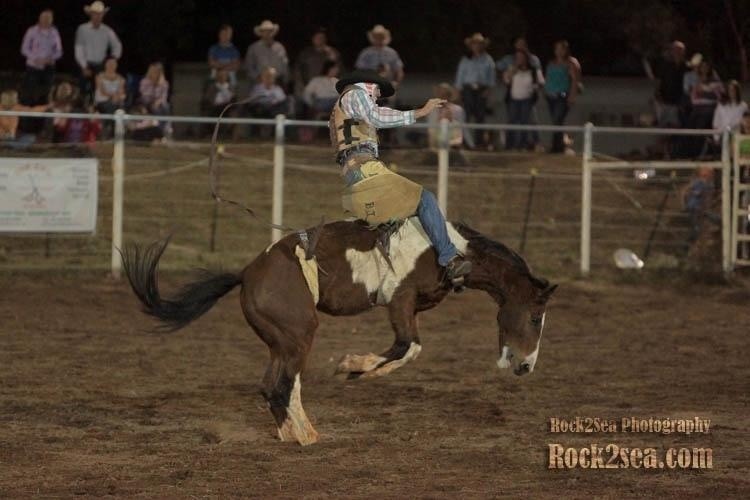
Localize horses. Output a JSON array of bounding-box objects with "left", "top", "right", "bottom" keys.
[{"left": 112, "top": 214, "right": 559, "bottom": 448}]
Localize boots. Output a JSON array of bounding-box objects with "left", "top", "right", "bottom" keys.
[{"left": 437, "top": 253, "right": 479, "bottom": 293}]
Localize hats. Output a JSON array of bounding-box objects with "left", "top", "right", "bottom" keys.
[
  {"left": 335, "top": 68, "right": 396, "bottom": 101},
  {"left": 432, "top": 83, "right": 458, "bottom": 103},
  {"left": 464, "top": 32, "right": 489, "bottom": 49},
  {"left": 82, "top": 1, "right": 112, "bottom": 15},
  {"left": 253, "top": 20, "right": 280, "bottom": 38},
  {"left": 49, "top": 81, "right": 80, "bottom": 107},
  {"left": 367, "top": 25, "right": 393, "bottom": 45}
]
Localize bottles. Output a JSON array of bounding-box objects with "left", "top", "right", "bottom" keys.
[{"left": 612, "top": 246, "right": 644, "bottom": 270}]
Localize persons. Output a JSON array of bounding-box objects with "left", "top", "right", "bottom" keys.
[
  {"left": 329, "top": 68, "right": 472, "bottom": 287},
  {"left": 0, "top": 0, "right": 749, "bottom": 188}
]
